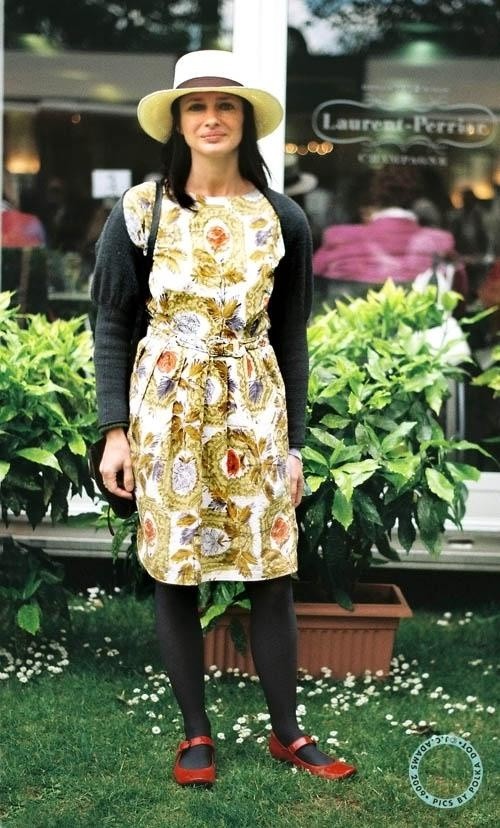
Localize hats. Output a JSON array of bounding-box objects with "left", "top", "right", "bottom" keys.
[{"left": 137, "top": 50, "right": 283, "bottom": 144}]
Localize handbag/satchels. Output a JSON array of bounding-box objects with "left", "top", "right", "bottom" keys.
[{"left": 89, "top": 438, "right": 137, "bottom": 518}]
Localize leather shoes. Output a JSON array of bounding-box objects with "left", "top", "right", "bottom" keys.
[
  {"left": 269, "top": 728, "right": 356, "bottom": 780},
  {"left": 174, "top": 736, "right": 217, "bottom": 787}
]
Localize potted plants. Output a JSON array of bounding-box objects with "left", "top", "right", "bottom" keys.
[{"left": 196, "top": 278, "right": 499, "bottom": 679}]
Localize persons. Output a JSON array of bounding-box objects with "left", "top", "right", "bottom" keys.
[
  {"left": 311, "top": 163, "right": 470, "bottom": 306},
  {"left": 88, "top": 47, "right": 359, "bottom": 788}
]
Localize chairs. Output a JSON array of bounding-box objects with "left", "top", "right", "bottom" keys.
[{"left": 318, "top": 279, "right": 465, "bottom": 463}]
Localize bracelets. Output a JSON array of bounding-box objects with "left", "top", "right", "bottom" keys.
[{"left": 289, "top": 449, "right": 303, "bottom": 462}]
